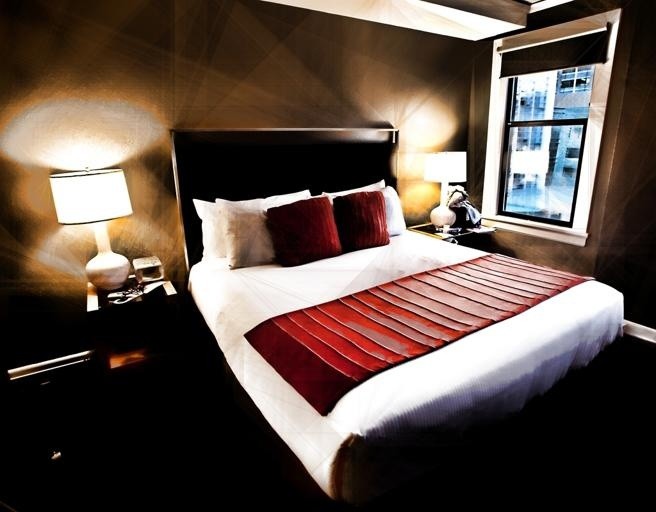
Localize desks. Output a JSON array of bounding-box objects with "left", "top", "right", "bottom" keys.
[{"left": 85, "top": 276, "right": 177, "bottom": 392}]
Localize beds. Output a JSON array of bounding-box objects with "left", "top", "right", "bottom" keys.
[{"left": 165, "top": 121, "right": 633, "bottom": 507}]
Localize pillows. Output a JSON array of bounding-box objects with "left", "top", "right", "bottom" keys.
[{"left": 188, "top": 177, "right": 407, "bottom": 270}]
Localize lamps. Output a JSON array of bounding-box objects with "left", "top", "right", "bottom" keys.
[
  {"left": 424, "top": 151, "right": 467, "bottom": 226},
  {"left": 48, "top": 167, "right": 137, "bottom": 291}
]
